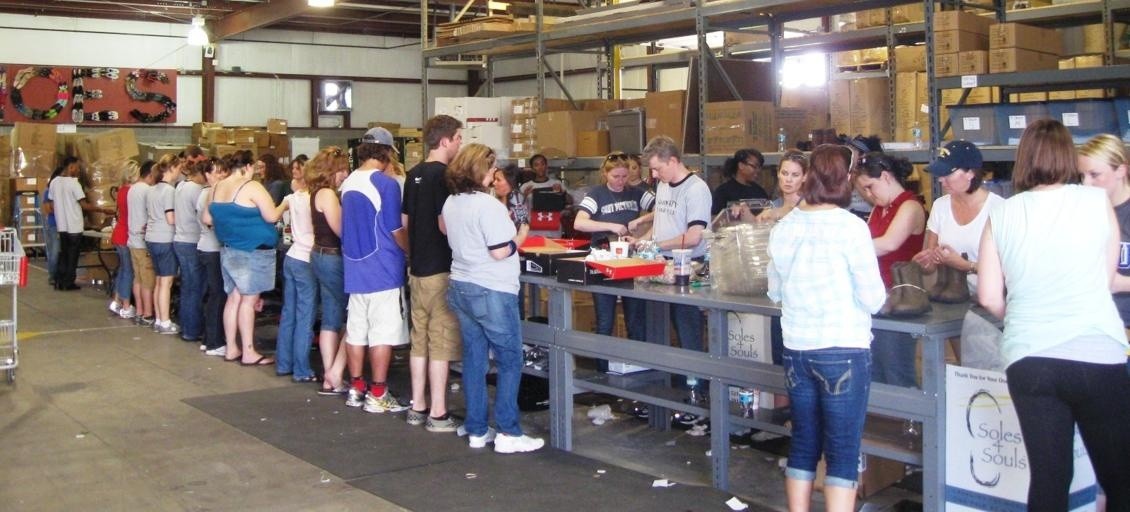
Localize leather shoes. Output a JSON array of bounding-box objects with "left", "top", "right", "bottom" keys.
[
  {"left": 59, "top": 282, "right": 81, "bottom": 291},
  {"left": 54, "top": 279, "right": 63, "bottom": 291}
]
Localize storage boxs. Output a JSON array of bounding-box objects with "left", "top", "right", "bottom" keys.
[
  {"left": 0, "top": 118, "right": 292, "bottom": 252},
  {"left": 404, "top": 140, "right": 424, "bottom": 172},
  {"left": 557, "top": 256, "right": 666, "bottom": 286},
  {"left": 907, "top": 164, "right": 919, "bottom": 182},
  {"left": 982, "top": 178, "right": 1014, "bottom": 199},
  {"left": 546, "top": 298, "right": 628, "bottom": 338},
  {"left": 517, "top": 234, "right": 591, "bottom": 278},
  {"left": 524, "top": 283, "right": 548, "bottom": 302},
  {"left": 524, "top": 295, "right": 546, "bottom": 320},
  {"left": 917, "top": 163, "right": 993, "bottom": 216}
]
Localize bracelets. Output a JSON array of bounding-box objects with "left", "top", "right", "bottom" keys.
[{"left": 966, "top": 262, "right": 977, "bottom": 275}]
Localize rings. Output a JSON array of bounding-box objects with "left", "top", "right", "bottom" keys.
[{"left": 935, "top": 257, "right": 942, "bottom": 262}]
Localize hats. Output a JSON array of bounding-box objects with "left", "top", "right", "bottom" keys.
[
  {"left": 357, "top": 126, "right": 401, "bottom": 155},
  {"left": 922, "top": 140, "right": 985, "bottom": 177}
]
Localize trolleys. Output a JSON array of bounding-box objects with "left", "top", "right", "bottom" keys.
[{"left": 1, "top": 227, "right": 28, "bottom": 384}]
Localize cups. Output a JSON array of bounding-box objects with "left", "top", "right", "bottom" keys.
[
  {"left": 609, "top": 241, "right": 629, "bottom": 259},
  {"left": 672, "top": 248, "right": 692, "bottom": 287}
]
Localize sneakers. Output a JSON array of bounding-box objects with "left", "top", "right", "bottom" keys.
[
  {"left": 468, "top": 426, "right": 498, "bottom": 449},
  {"left": 199, "top": 344, "right": 207, "bottom": 352},
  {"left": 158, "top": 321, "right": 183, "bottom": 335},
  {"left": 107, "top": 300, "right": 124, "bottom": 316},
  {"left": 344, "top": 382, "right": 369, "bottom": 408},
  {"left": 636, "top": 403, "right": 649, "bottom": 419},
  {"left": 406, "top": 407, "right": 430, "bottom": 426},
  {"left": 47, "top": 277, "right": 56, "bottom": 285},
  {"left": 152, "top": 321, "right": 160, "bottom": 333},
  {"left": 132, "top": 314, "right": 144, "bottom": 326},
  {"left": 362, "top": 385, "right": 414, "bottom": 414},
  {"left": 119, "top": 304, "right": 137, "bottom": 320},
  {"left": 205, "top": 344, "right": 227, "bottom": 356},
  {"left": 677, "top": 412, "right": 707, "bottom": 425},
  {"left": 423, "top": 413, "right": 465, "bottom": 433},
  {"left": 138, "top": 315, "right": 156, "bottom": 329},
  {"left": 492, "top": 431, "right": 545, "bottom": 454}
]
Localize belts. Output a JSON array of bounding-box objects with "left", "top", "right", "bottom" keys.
[{"left": 312, "top": 244, "right": 342, "bottom": 255}]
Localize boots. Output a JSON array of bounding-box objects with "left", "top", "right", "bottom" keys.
[
  {"left": 926, "top": 261, "right": 950, "bottom": 302},
  {"left": 874, "top": 260, "right": 909, "bottom": 320},
  {"left": 936, "top": 252, "right": 971, "bottom": 305},
  {"left": 890, "top": 260, "right": 934, "bottom": 321}
]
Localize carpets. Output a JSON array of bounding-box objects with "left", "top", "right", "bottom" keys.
[
  {"left": 179, "top": 380, "right": 497, "bottom": 482},
  {"left": 345, "top": 444, "right": 775, "bottom": 512}
]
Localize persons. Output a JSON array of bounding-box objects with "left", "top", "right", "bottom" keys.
[
  {"left": 41, "top": 159, "right": 116, "bottom": 291},
  {"left": 110, "top": 116, "right": 576, "bottom": 455},
  {"left": 911, "top": 141, "right": 1007, "bottom": 363},
  {"left": 847, "top": 135, "right": 882, "bottom": 219},
  {"left": 731, "top": 150, "right": 807, "bottom": 443},
  {"left": 575, "top": 135, "right": 712, "bottom": 425},
  {"left": 976, "top": 118, "right": 1130, "bottom": 512},
  {"left": 1074, "top": 132, "right": 1130, "bottom": 351},
  {"left": 852, "top": 151, "right": 925, "bottom": 388},
  {"left": 767, "top": 144, "right": 886, "bottom": 512},
  {"left": 713, "top": 149, "right": 769, "bottom": 220}
]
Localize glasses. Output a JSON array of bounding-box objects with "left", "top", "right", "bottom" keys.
[{"left": 603, "top": 154, "right": 628, "bottom": 169}]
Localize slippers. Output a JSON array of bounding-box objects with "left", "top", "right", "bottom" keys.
[
  {"left": 223, "top": 354, "right": 242, "bottom": 362},
  {"left": 239, "top": 355, "right": 277, "bottom": 367},
  {"left": 316, "top": 383, "right": 350, "bottom": 397}
]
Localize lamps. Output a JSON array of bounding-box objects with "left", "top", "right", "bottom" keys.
[{"left": 188, "top": 0, "right": 208, "bottom": 46}]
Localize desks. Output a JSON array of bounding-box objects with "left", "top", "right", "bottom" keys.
[
  {"left": 519, "top": 272, "right": 1004, "bottom": 511},
  {"left": 82, "top": 229, "right": 120, "bottom": 297}
]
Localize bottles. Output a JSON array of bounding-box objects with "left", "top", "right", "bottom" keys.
[
  {"left": 687, "top": 376, "right": 705, "bottom": 406},
  {"left": 111, "top": 217, "right": 119, "bottom": 232},
  {"left": 739, "top": 388, "right": 755, "bottom": 417},
  {"left": 777, "top": 126, "right": 786, "bottom": 153},
  {"left": 914, "top": 121, "right": 922, "bottom": 145}
]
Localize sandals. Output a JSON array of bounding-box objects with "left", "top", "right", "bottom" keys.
[
  {"left": 290, "top": 371, "right": 324, "bottom": 383},
  {"left": 276, "top": 370, "right": 294, "bottom": 377}
]
